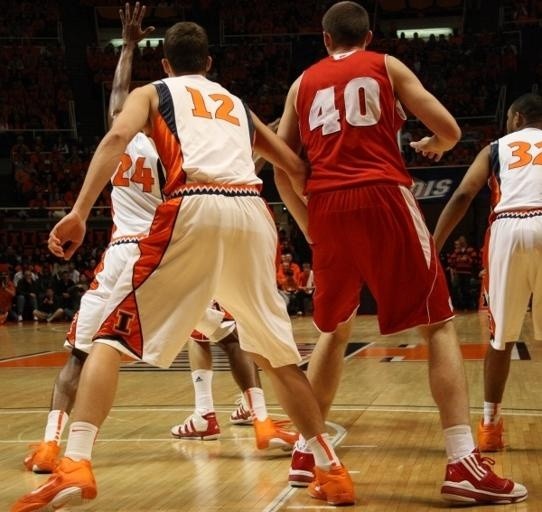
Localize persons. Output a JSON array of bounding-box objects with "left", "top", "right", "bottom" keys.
[
  {"left": 0, "top": 0, "right": 542, "bottom": 322},
  {"left": 272, "top": 0, "right": 527, "bottom": 506},
  {"left": 172, "top": 119, "right": 282, "bottom": 439},
  {"left": 6, "top": 19, "right": 356, "bottom": 512},
  {"left": 22, "top": 0, "right": 300, "bottom": 475},
  {"left": 430, "top": 93, "right": 542, "bottom": 452}
]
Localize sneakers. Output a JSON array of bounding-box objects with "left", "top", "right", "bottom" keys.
[
  {"left": 11, "top": 457, "right": 98, "bottom": 511},
  {"left": 477, "top": 415, "right": 505, "bottom": 453},
  {"left": 253, "top": 417, "right": 298, "bottom": 452},
  {"left": 23, "top": 441, "right": 62, "bottom": 473},
  {"left": 229, "top": 403, "right": 256, "bottom": 425},
  {"left": 171, "top": 410, "right": 221, "bottom": 441},
  {"left": 289, "top": 440, "right": 315, "bottom": 487},
  {"left": 440, "top": 448, "right": 529, "bottom": 506},
  {"left": 306, "top": 463, "right": 357, "bottom": 506}
]
